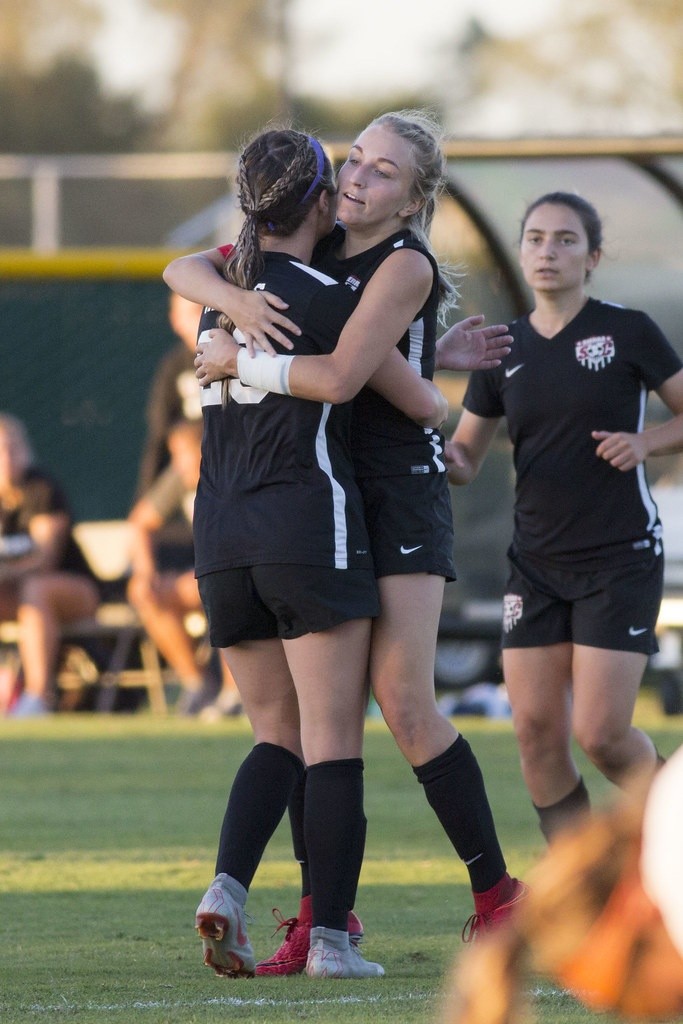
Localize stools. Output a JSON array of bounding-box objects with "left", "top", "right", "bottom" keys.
[
  {"left": 0, "top": 602, "right": 100, "bottom": 709},
  {"left": 95, "top": 602, "right": 180, "bottom": 716}
]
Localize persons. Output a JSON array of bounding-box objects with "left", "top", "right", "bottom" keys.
[
  {"left": 161, "top": 110, "right": 532, "bottom": 975},
  {"left": 191, "top": 127, "right": 516, "bottom": 978},
  {"left": 433, "top": 741, "right": 682, "bottom": 1023},
  {"left": 0, "top": 415, "right": 106, "bottom": 717},
  {"left": 436, "top": 189, "right": 682, "bottom": 848},
  {"left": 125, "top": 416, "right": 244, "bottom": 723}
]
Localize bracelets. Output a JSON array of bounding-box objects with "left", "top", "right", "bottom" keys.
[{"left": 238, "top": 344, "right": 294, "bottom": 399}]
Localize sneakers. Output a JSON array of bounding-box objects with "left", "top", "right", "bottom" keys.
[
  {"left": 254, "top": 907, "right": 365, "bottom": 977},
  {"left": 195, "top": 886, "right": 255, "bottom": 978},
  {"left": 304, "top": 926, "right": 386, "bottom": 980},
  {"left": 460, "top": 879, "right": 528, "bottom": 952}
]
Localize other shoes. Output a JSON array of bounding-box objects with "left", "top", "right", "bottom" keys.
[
  {"left": 11, "top": 692, "right": 59, "bottom": 718},
  {"left": 169, "top": 673, "right": 217, "bottom": 717}
]
